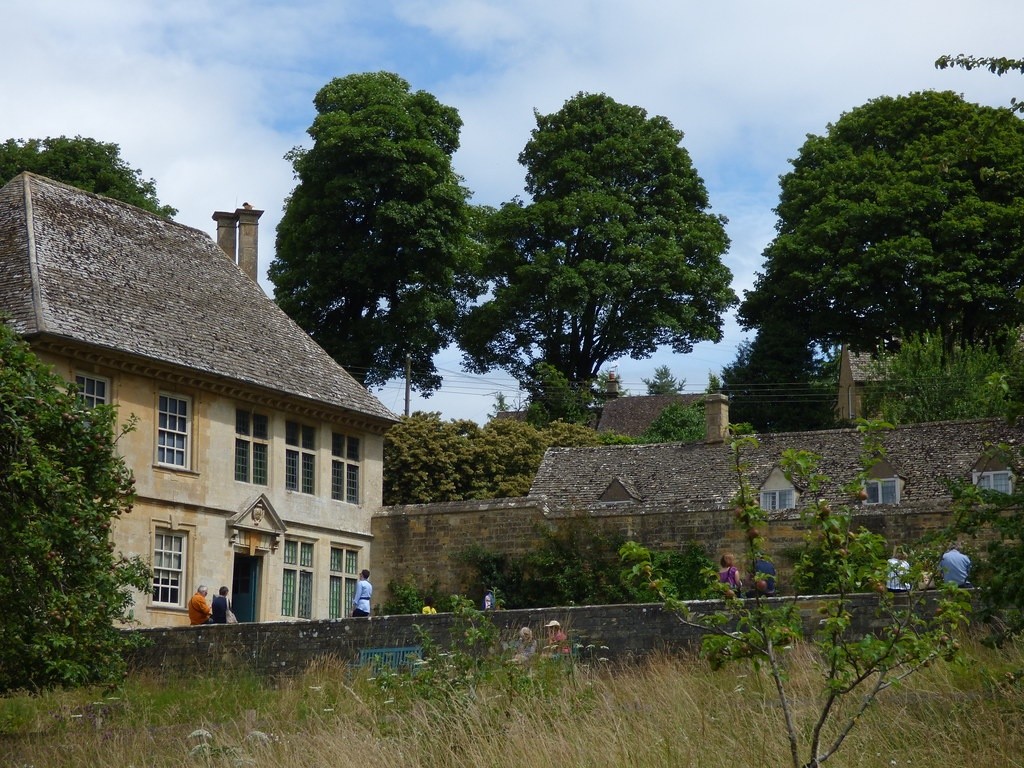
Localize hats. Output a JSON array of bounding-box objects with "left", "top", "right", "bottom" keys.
[{"left": 545, "top": 620, "right": 560, "bottom": 626}]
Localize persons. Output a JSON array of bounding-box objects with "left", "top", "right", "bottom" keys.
[
  {"left": 352, "top": 570, "right": 373, "bottom": 617},
  {"left": 938, "top": 541, "right": 972, "bottom": 588},
  {"left": 212, "top": 587, "right": 231, "bottom": 623},
  {"left": 545, "top": 620, "right": 571, "bottom": 653},
  {"left": 744, "top": 551, "right": 776, "bottom": 597},
  {"left": 887, "top": 551, "right": 911, "bottom": 591},
  {"left": 422, "top": 597, "right": 437, "bottom": 614},
  {"left": 188, "top": 586, "right": 213, "bottom": 624},
  {"left": 483, "top": 591, "right": 495, "bottom": 610},
  {"left": 513, "top": 627, "right": 537, "bottom": 659},
  {"left": 719, "top": 553, "right": 743, "bottom": 598}
]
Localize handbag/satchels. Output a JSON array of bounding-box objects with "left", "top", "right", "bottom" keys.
[
  {"left": 226, "top": 598, "right": 238, "bottom": 624},
  {"left": 918, "top": 571, "right": 937, "bottom": 590}
]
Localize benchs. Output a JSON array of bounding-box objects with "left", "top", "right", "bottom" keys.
[
  {"left": 355, "top": 647, "right": 426, "bottom": 684},
  {"left": 554, "top": 638, "right": 583, "bottom": 660}
]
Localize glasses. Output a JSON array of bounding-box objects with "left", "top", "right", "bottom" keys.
[
  {"left": 360, "top": 573, "right": 363, "bottom": 575},
  {"left": 204, "top": 592, "right": 207, "bottom": 596}
]
